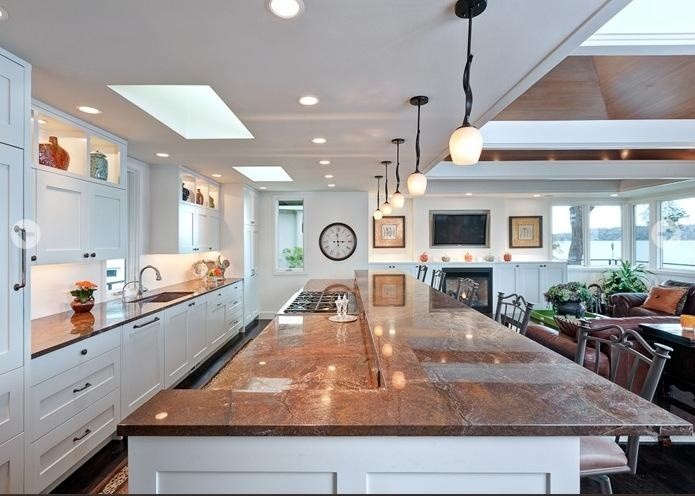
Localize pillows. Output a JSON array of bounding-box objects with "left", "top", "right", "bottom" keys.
[
  {"left": 552, "top": 316, "right": 592, "bottom": 340},
  {"left": 640, "top": 283, "right": 687, "bottom": 316},
  {"left": 658, "top": 284, "right": 688, "bottom": 315}
]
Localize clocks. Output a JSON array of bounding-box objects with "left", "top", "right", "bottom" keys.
[{"left": 318, "top": 222, "right": 357, "bottom": 261}]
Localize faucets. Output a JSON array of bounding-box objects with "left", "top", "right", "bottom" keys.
[
  {"left": 121, "top": 279, "right": 139, "bottom": 304},
  {"left": 136, "top": 265, "right": 161, "bottom": 299}
]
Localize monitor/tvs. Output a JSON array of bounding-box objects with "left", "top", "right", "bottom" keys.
[{"left": 429, "top": 210, "right": 491, "bottom": 250}]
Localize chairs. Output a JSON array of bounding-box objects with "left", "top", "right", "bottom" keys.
[
  {"left": 431, "top": 270, "right": 445, "bottom": 291},
  {"left": 416, "top": 264, "right": 427, "bottom": 283},
  {"left": 456, "top": 277, "right": 479, "bottom": 309},
  {"left": 579, "top": 329, "right": 674, "bottom": 496},
  {"left": 572, "top": 326, "right": 632, "bottom": 387},
  {"left": 494, "top": 291, "right": 535, "bottom": 337}
]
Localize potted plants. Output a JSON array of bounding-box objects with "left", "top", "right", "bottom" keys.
[{"left": 599, "top": 258, "right": 656, "bottom": 318}]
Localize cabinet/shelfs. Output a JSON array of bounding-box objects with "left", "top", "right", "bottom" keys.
[
  {"left": 121, "top": 309, "right": 164, "bottom": 421},
  {"left": 31, "top": 98, "right": 130, "bottom": 266},
  {"left": 30, "top": 326, "right": 121, "bottom": 494},
  {"left": 210, "top": 277, "right": 243, "bottom": 357},
  {"left": 164, "top": 294, "right": 210, "bottom": 389},
  {"left": 494, "top": 263, "right": 519, "bottom": 315},
  {"left": 519, "top": 260, "right": 568, "bottom": 313},
  {"left": 150, "top": 164, "right": 220, "bottom": 254},
  {"left": 221, "top": 183, "right": 258, "bottom": 331}
]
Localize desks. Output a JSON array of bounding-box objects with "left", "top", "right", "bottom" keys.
[{"left": 636, "top": 320, "right": 695, "bottom": 446}]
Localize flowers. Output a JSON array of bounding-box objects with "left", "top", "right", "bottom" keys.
[
  {"left": 71, "top": 281, "right": 98, "bottom": 302},
  {"left": 542, "top": 279, "right": 598, "bottom": 319},
  {"left": 209, "top": 267, "right": 223, "bottom": 276}
]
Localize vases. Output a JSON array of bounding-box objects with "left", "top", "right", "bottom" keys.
[
  {"left": 195, "top": 188, "right": 203, "bottom": 205},
  {"left": 212, "top": 275, "right": 216, "bottom": 281},
  {"left": 39, "top": 135, "right": 69, "bottom": 173},
  {"left": 71, "top": 295, "right": 93, "bottom": 313},
  {"left": 181, "top": 183, "right": 190, "bottom": 201},
  {"left": 558, "top": 301, "right": 582, "bottom": 318}
]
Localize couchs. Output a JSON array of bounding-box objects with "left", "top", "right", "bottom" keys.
[
  {"left": 524, "top": 314, "right": 686, "bottom": 399},
  {"left": 610, "top": 279, "right": 694, "bottom": 317}
]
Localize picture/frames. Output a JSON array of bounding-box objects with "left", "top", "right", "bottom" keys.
[
  {"left": 509, "top": 215, "right": 543, "bottom": 248},
  {"left": 372, "top": 273, "right": 405, "bottom": 308},
  {"left": 372, "top": 216, "right": 405, "bottom": 249}
]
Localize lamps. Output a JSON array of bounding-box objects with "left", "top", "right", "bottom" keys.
[
  {"left": 389, "top": 138, "right": 405, "bottom": 210},
  {"left": 380, "top": 161, "right": 392, "bottom": 215},
  {"left": 373, "top": 176, "right": 383, "bottom": 221},
  {"left": 406, "top": 95, "right": 428, "bottom": 195},
  {"left": 448, "top": 1, "right": 486, "bottom": 167}
]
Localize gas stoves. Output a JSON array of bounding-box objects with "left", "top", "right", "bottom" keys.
[{"left": 278, "top": 291, "right": 356, "bottom": 315}]
